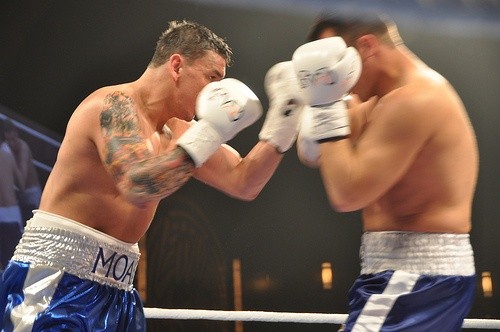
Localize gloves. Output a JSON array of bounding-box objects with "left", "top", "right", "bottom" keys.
[
  {"left": 177, "top": 78, "right": 263, "bottom": 170},
  {"left": 292, "top": 36, "right": 357, "bottom": 141},
  {"left": 258, "top": 60, "right": 306, "bottom": 153}
]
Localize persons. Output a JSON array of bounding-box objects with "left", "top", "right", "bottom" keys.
[
  {"left": 0, "top": 120, "right": 43, "bottom": 269},
  {"left": 0, "top": 21, "right": 307, "bottom": 332},
  {"left": 295, "top": 5, "right": 479, "bottom": 332}
]
